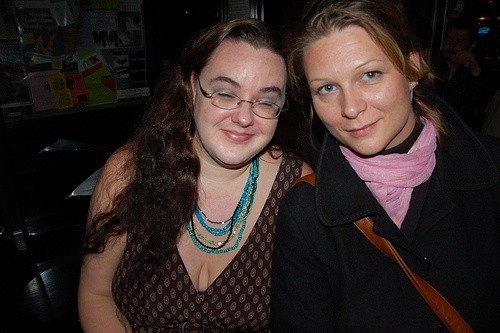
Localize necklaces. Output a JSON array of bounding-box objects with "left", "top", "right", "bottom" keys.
[{"left": 185, "top": 156, "right": 260, "bottom": 256}]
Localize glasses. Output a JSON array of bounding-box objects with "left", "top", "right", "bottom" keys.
[{"left": 196, "top": 76, "right": 289, "bottom": 120}]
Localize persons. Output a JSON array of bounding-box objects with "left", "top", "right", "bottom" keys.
[
  {"left": 274, "top": 1, "right": 500, "bottom": 332},
  {"left": 77, "top": 18, "right": 317, "bottom": 333},
  {"left": 418, "top": 17, "right": 485, "bottom": 126}
]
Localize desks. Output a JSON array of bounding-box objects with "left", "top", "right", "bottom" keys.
[{"left": 1, "top": 98, "right": 151, "bottom": 200}]
